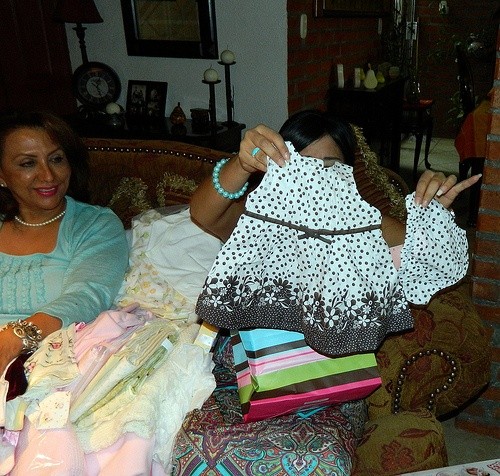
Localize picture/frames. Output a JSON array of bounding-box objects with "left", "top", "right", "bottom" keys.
[
  {"left": 312, "top": 0, "right": 392, "bottom": 18},
  {"left": 120, "top": 0, "right": 219, "bottom": 59},
  {"left": 126, "top": 79, "right": 168, "bottom": 119}
]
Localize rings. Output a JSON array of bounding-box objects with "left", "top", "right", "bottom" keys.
[{"left": 251, "top": 146, "right": 261, "bottom": 157}]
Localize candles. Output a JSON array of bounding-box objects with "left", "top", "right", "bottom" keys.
[
  {"left": 205, "top": 64, "right": 217, "bottom": 82},
  {"left": 220, "top": 45, "right": 234, "bottom": 64}
]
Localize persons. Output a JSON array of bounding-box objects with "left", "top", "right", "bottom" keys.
[
  {"left": 172, "top": 104, "right": 483, "bottom": 476},
  {"left": 0, "top": 110, "right": 130, "bottom": 476}
]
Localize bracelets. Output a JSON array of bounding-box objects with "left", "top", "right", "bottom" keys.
[
  {"left": 212, "top": 157, "right": 249, "bottom": 200},
  {"left": 0, "top": 318, "right": 43, "bottom": 355}
]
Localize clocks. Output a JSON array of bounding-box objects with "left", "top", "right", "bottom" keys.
[{"left": 72, "top": 61, "right": 122, "bottom": 107}]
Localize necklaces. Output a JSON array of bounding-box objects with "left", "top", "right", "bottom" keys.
[{"left": 14, "top": 210, "right": 66, "bottom": 227}]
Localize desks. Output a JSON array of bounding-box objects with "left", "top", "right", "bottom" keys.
[
  {"left": 329, "top": 71, "right": 441, "bottom": 171},
  {"left": 456, "top": 99, "right": 493, "bottom": 229},
  {"left": 59, "top": 113, "right": 245, "bottom": 154}
]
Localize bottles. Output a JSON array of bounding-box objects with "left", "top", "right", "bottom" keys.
[{"left": 169, "top": 102, "right": 186, "bottom": 125}]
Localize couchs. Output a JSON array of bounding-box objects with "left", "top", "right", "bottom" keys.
[{"left": 81, "top": 138, "right": 491, "bottom": 476}]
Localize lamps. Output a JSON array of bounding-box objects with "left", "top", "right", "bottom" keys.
[{"left": 50, "top": 0, "right": 103, "bottom": 65}]
